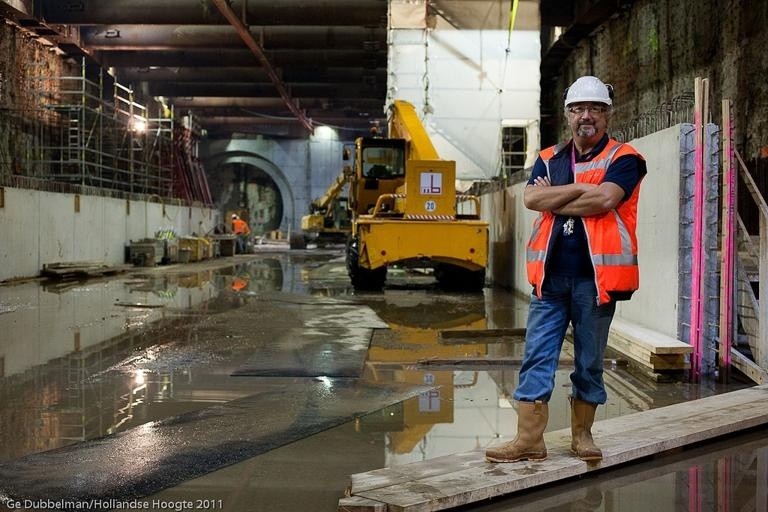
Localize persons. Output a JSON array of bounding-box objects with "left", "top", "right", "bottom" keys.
[
  {"left": 230, "top": 214, "right": 246, "bottom": 255},
  {"left": 237, "top": 216, "right": 250, "bottom": 254},
  {"left": 485, "top": 74, "right": 645, "bottom": 461}
]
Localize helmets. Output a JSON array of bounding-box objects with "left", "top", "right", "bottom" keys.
[
  {"left": 231, "top": 213, "right": 240, "bottom": 219},
  {"left": 564, "top": 76, "right": 612, "bottom": 107}
]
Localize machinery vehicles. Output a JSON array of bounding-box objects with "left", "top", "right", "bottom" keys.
[
  {"left": 287, "top": 165, "right": 354, "bottom": 250},
  {"left": 339, "top": 99, "right": 492, "bottom": 290},
  {"left": 348, "top": 283, "right": 494, "bottom": 460}
]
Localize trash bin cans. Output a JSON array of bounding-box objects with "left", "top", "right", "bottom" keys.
[{"left": 179, "top": 247, "right": 191, "bottom": 264}]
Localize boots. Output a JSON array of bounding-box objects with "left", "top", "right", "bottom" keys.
[
  {"left": 569, "top": 396, "right": 602, "bottom": 460},
  {"left": 486, "top": 400, "right": 548, "bottom": 463}
]
